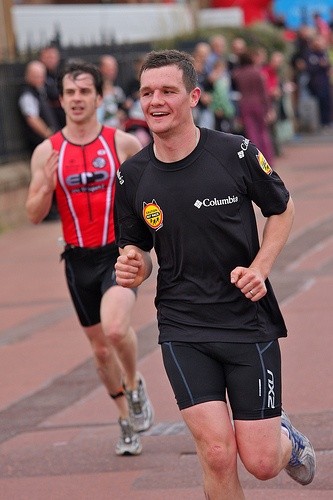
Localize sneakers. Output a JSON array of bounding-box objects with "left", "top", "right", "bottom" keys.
[
  {"left": 115, "top": 416, "right": 143, "bottom": 456},
  {"left": 121, "top": 371, "right": 155, "bottom": 433},
  {"left": 279, "top": 408, "right": 316, "bottom": 486}
]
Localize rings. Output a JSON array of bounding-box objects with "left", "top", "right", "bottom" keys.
[{"left": 248, "top": 290, "right": 255, "bottom": 296}]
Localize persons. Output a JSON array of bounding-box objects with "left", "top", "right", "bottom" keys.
[
  {"left": 16, "top": 12, "right": 333, "bottom": 224},
  {"left": 26, "top": 61, "right": 154, "bottom": 457},
  {"left": 113, "top": 51, "right": 317, "bottom": 500}
]
